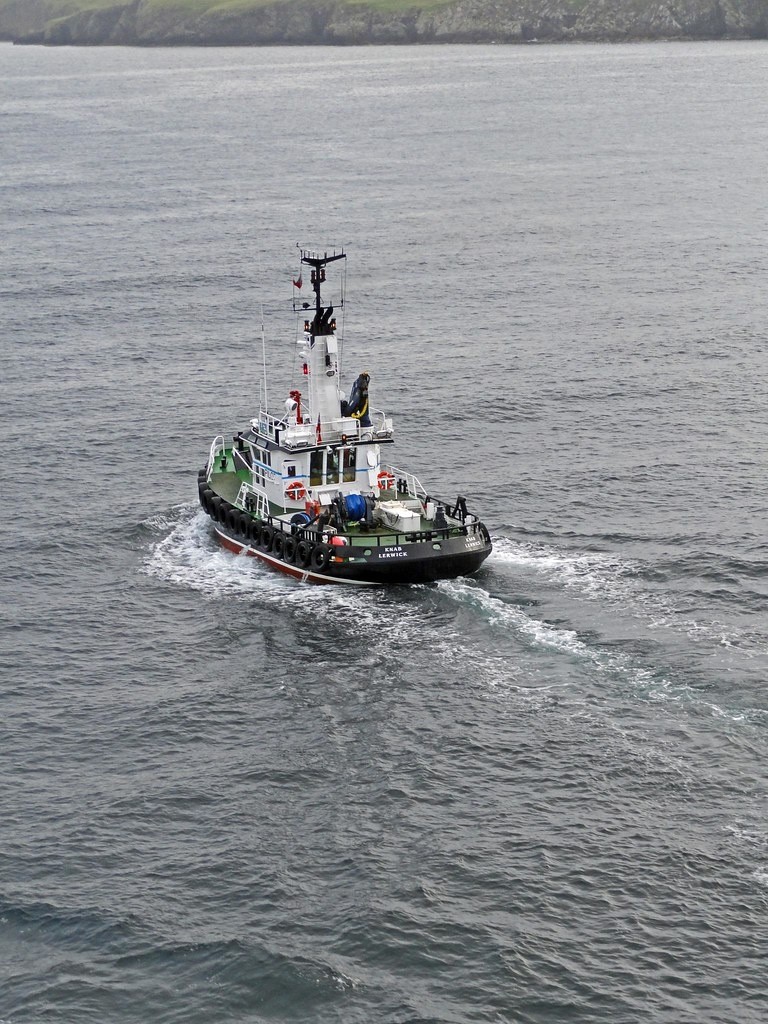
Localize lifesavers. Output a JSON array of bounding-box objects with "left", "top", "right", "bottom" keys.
[
  {"left": 377, "top": 471, "right": 393, "bottom": 490},
  {"left": 288, "top": 482, "right": 305, "bottom": 499},
  {"left": 197, "top": 469, "right": 331, "bottom": 572}
]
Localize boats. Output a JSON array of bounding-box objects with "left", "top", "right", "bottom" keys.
[{"left": 197, "top": 241, "right": 492, "bottom": 588}]
